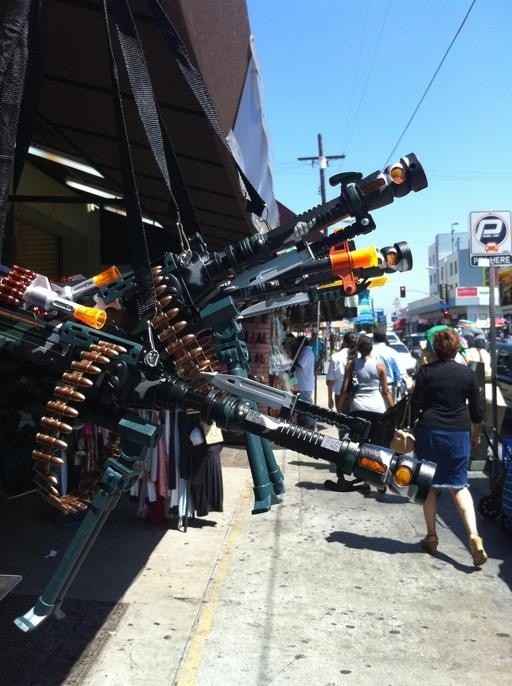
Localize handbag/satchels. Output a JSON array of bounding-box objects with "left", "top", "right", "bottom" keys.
[
  {"left": 350, "top": 359, "right": 359, "bottom": 391},
  {"left": 390, "top": 428, "right": 416, "bottom": 455}
]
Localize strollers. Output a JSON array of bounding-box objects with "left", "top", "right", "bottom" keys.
[{"left": 478, "top": 422, "right": 511, "bottom": 536}]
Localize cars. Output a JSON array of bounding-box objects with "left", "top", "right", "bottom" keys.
[{"left": 364, "top": 330, "right": 416, "bottom": 387}]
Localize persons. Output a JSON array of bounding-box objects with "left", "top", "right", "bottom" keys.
[
  {"left": 283, "top": 323, "right": 512, "bottom": 490},
  {"left": 411, "top": 328, "right": 487, "bottom": 566}
]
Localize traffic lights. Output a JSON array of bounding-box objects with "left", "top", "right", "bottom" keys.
[{"left": 399, "top": 285, "right": 405, "bottom": 298}]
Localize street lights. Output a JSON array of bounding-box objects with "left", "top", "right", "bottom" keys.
[{"left": 424, "top": 265, "right": 447, "bottom": 305}]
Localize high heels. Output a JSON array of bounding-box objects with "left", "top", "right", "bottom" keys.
[
  {"left": 469, "top": 537, "right": 487, "bottom": 566},
  {"left": 423, "top": 534, "right": 439, "bottom": 553}
]
